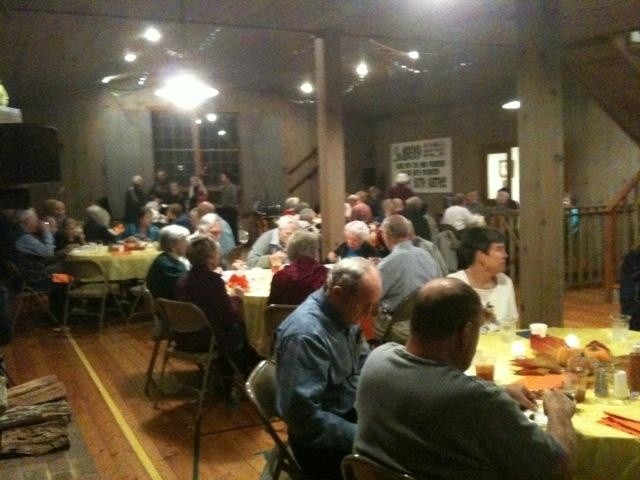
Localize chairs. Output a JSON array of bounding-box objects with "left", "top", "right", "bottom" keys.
[
  {"left": 2, "top": 196, "right": 238, "bottom": 330},
  {"left": 245, "top": 357, "right": 308, "bottom": 480},
  {"left": 340, "top": 452, "right": 415, "bottom": 478},
  {"left": 141, "top": 285, "right": 426, "bottom": 422}
]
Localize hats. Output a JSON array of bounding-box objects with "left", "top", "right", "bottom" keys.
[{"left": 395, "top": 173, "right": 411, "bottom": 183}]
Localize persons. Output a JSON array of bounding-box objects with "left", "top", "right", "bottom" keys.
[
  {"left": 145, "top": 172, "right": 516, "bottom": 396},
  {"left": 276, "top": 225, "right": 579, "bottom": 478},
  {"left": 1, "top": 165, "right": 238, "bottom": 332},
  {"left": 620, "top": 244, "right": 640, "bottom": 397},
  {"left": 564, "top": 195, "right": 580, "bottom": 256}
]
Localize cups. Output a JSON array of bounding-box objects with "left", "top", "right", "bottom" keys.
[
  {"left": 472, "top": 356, "right": 495, "bottom": 379},
  {"left": 568, "top": 365, "right": 588, "bottom": 402},
  {"left": 88, "top": 242, "right": 97, "bottom": 251},
  {"left": 270, "top": 256, "right": 283, "bottom": 273},
  {"left": 498, "top": 317, "right": 518, "bottom": 343},
  {"left": 529, "top": 322, "right": 548, "bottom": 338},
  {"left": 609, "top": 313, "right": 632, "bottom": 342}
]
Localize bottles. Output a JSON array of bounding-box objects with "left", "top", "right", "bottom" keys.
[
  {"left": 612, "top": 370, "right": 630, "bottom": 399},
  {"left": 595, "top": 370, "right": 608, "bottom": 398}
]
